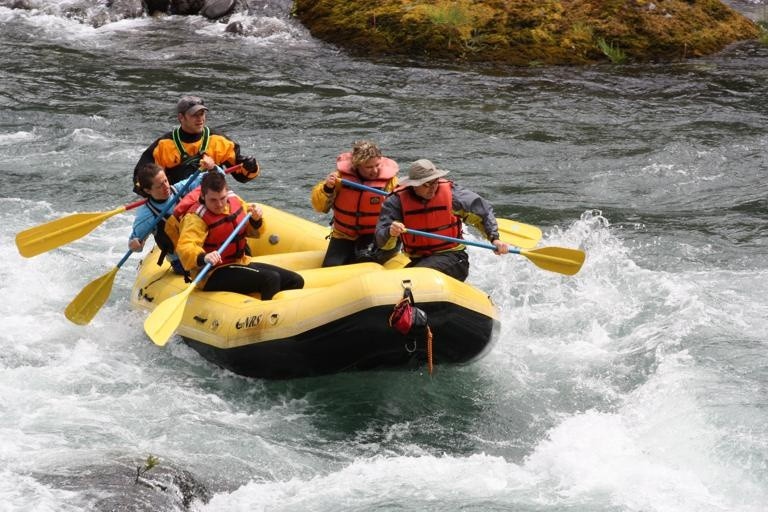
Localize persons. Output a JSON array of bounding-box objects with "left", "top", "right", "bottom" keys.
[
  {"left": 173, "top": 169, "right": 305, "bottom": 302},
  {"left": 309, "top": 141, "right": 400, "bottom": 268},
  {"left": 129, "top": 95, "right": 259, "bottom": 196},
  {"left": 376, "top": 159, "right": 509, "bottom": 283},
  {"left": 122, "top": 155, "right": 225, "bottom": 276}
]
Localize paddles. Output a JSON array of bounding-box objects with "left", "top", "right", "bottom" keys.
[
  {"left": 145, "top": 210, "right": 252, "bottom": 347},
  {"left": 400, "top": 228, "right": 585, "bottom": 274},
  {"left": 16, "top": 162, "right": 246, "bottom": 258},
  {"left": 337, "top": 178, "right": 542, "bottom": 251},
  {"left": 64, "top": 160, "right": 208, "bottom": 324}
]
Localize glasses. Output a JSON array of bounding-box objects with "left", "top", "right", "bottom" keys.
[{"left": 423, "top": 177, "right": 440, "bottom": 187}]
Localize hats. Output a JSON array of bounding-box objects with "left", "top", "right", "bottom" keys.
[
  {"left": 395, "top": 158, "right": 451, "bottom": 188},
  {"left": 175, "top": 95, "right": 210, "bottom": 116}
]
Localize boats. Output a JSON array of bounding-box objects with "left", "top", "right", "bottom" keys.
[{"left": 130, "top": 201, "right": 501, "bottom": 383}]
